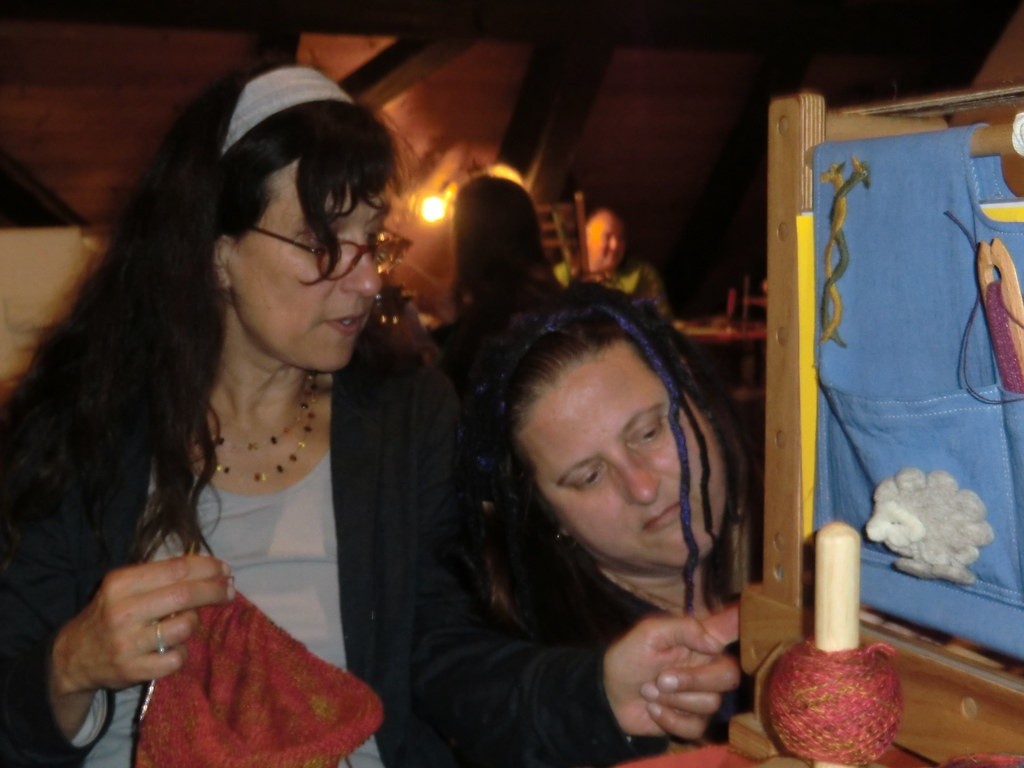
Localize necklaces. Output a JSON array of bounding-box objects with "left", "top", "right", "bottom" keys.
[{"left": 198, "top": 372, "right": 324, "bottom": 483}]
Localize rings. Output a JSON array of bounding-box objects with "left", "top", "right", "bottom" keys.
[{"left": 156, "top": 622, "right": 162, "bottom": 648}]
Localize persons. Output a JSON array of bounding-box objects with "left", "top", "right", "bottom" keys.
[
  {"left": 0, "top": 60, "right": 743, "bottom": 768},
  {"left": 555, "top": 209, "right": 666, "bottom": 314},
  {"left": 462, "top": 282, "right": 759, "bottom": 635},
  {"left": 431, "top": 177, "right": 564, "bottom": 364}
]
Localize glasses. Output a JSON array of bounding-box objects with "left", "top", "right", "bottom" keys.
[{"left": 251, "top": 224, "right": 413, "bottom": 281}]
